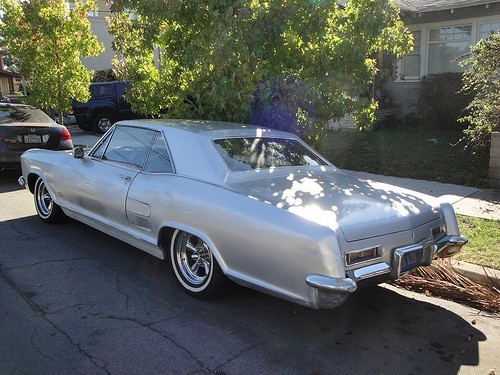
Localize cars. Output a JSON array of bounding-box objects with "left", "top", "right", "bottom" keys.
[
  {"left": 0, "top": 94, "right": 28, "bottom": 103},
  {"left": 0, "top": 102, "right": 74, "bottom": 177},
  {"left": 20, "top": 118, "right": 469, "bottom": 309}
]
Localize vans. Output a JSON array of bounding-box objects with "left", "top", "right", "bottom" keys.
[{"left": 71, "top": 79, "right": 159, "bottom": 132}]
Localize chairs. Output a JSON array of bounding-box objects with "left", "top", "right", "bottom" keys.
[{"left": 134, "top": 148, "right": 171, "bottom": 174}]
